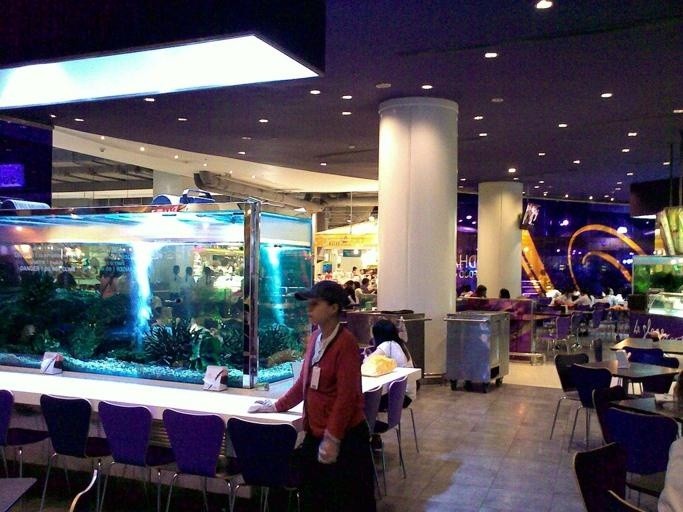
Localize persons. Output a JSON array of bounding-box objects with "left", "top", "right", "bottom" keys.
[
  {"left": 250, "top": 282, "right": 377, "bottom": 512},
  {"left": 365, "top": 319, "right": 416, "bottom": 412},
  {"left": 458, "top": 285, "right": 629, "bottom": 339},
  {"left": 324, "top": 262, "right": 377, "bottom": 309},
  {"left": 657, "top": 372, "right": 683, "bottom": 512},
  {"left": 57, "top": 256, "right": 244, "bottom": 300}
]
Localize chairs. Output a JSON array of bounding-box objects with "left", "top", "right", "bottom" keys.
[
  {"left": 527, "top": 294, "right": 682, "bottom": 512},
  {"left": 363, "top": 376, "right": 420, "bottom": 501},
  {"left": 311, "top": 297, "right": 359, "bottom": 331},
  {"left": 1, "top": 390, "right": 298, "bottom": 512}
]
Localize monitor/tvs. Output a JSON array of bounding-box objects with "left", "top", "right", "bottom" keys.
[
  {"left": 342, "top": 250, "right": 361, "bottom": 257},
  {"left": 519, "top": 202, "right": 542, "bottom": 230}
]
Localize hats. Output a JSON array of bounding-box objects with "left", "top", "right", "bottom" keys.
[{"left": 294, "top": 280, "right": 346, "bottom": 304}]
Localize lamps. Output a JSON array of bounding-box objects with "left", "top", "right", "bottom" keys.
[
  {"left": 0, "top": 1, "right": 329, "bottom": 111},
  {"left": 629, "top": 141, "right": 683, "bottom": 220}
]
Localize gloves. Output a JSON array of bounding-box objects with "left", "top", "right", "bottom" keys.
[
  {"left": 246, "top": 399, "right": 278, "bottom": 413},
  {"left": 318, "top": 428, "right": 341, "bottom": 464}
]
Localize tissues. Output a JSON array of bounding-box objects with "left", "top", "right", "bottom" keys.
[
  {"left": 202, "top": 365, "right": 228, "bottom": 392},
  {"left": 40, "top": 351, "right": 63, "bottom": 375}
]
